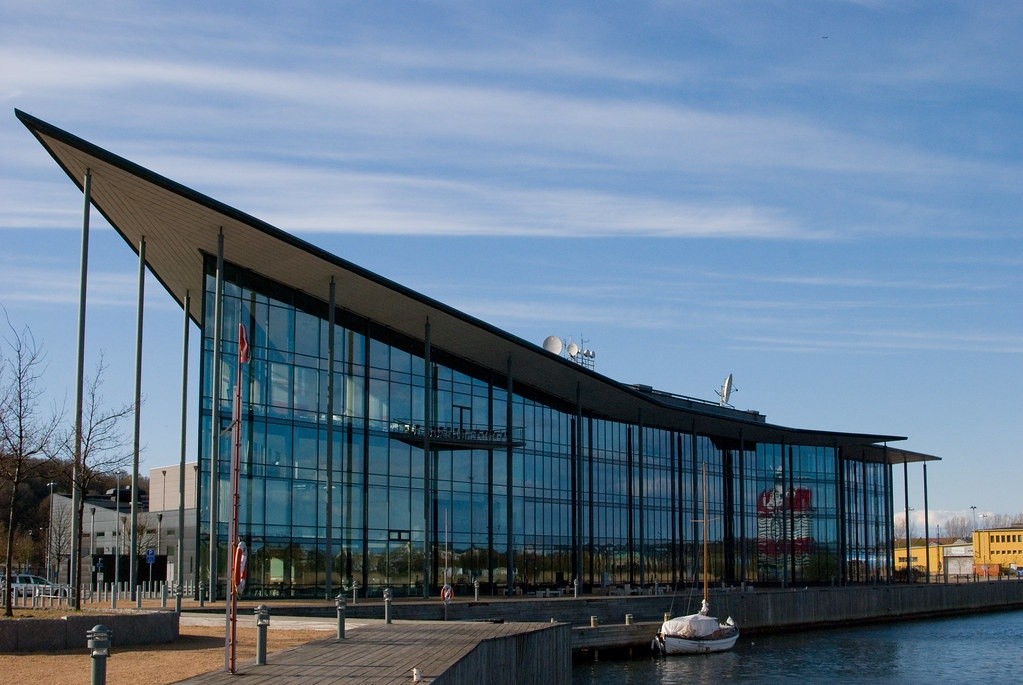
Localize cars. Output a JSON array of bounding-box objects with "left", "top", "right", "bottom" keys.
[{"left": 0, "top": 573, "right": 71, "bottom": 599}]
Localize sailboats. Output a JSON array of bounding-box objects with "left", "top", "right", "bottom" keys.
[{"left": 650, "top": 460, "right": 748, "bottom": 660}]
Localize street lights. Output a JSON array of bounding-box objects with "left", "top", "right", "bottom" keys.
[
  {"left": 969, "top": 505, "right": 978, "bottom": 577},
  {"left": 46, "top": 480, "right": 58, "bottom": 580},
  {"left": 978, "top": 514, "right": 989, "bottom": 578}
]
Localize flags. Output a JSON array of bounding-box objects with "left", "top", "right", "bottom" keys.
[{"left": 240, "top": 323, "right": 251, "bottom": 365}]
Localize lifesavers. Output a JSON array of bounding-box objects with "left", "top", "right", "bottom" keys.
[
  {"left": 441, "top": 585, "right": 453, "bottom": 602},
  {"left": 234, "top": 541, "right": 248, "bottom": 595}
]
